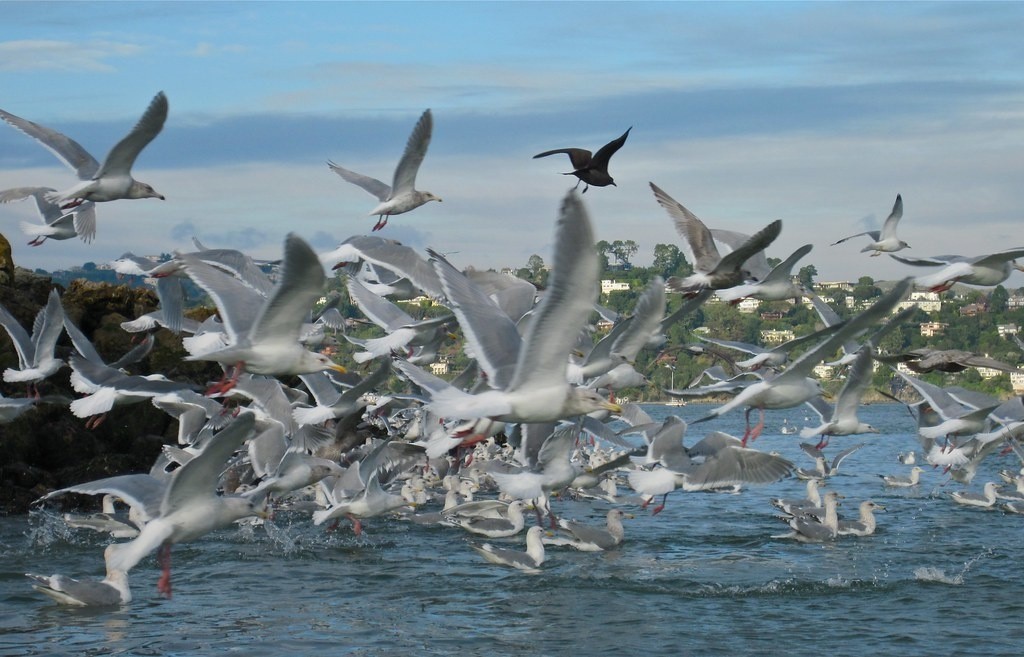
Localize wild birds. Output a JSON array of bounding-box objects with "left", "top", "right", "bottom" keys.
[{"left": 0, "top": 92, "right": 1024, "bottom": 604}]
